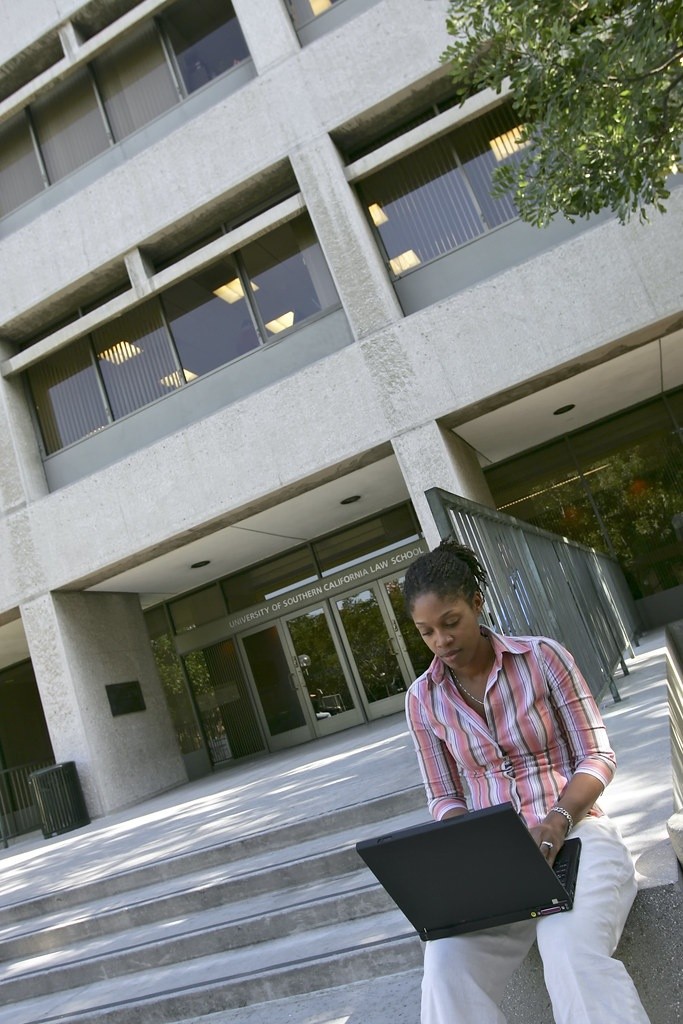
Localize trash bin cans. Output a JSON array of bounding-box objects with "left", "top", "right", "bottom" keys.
[{"left": 28, "top": 761, "right": 90, "bottom": 839}]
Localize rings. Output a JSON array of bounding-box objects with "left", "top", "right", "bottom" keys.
[{"left": 542, "top": 842, "right": 554, "bottom": 848}]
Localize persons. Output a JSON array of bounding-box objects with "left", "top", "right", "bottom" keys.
[{"left": 404, "top": 536, "right": 651, "bottom": 1024}]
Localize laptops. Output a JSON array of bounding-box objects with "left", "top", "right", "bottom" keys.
[{"left": 356, "top": 801, "right": 581, "bottom": 942}]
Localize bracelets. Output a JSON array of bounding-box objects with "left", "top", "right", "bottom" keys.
[{"left": 551, "top": 807, "right": 574, "bottom": 837}]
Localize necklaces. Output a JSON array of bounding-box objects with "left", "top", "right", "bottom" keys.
[{"left": 450, "top": 670, "right": 484, "bottom": 705}]
